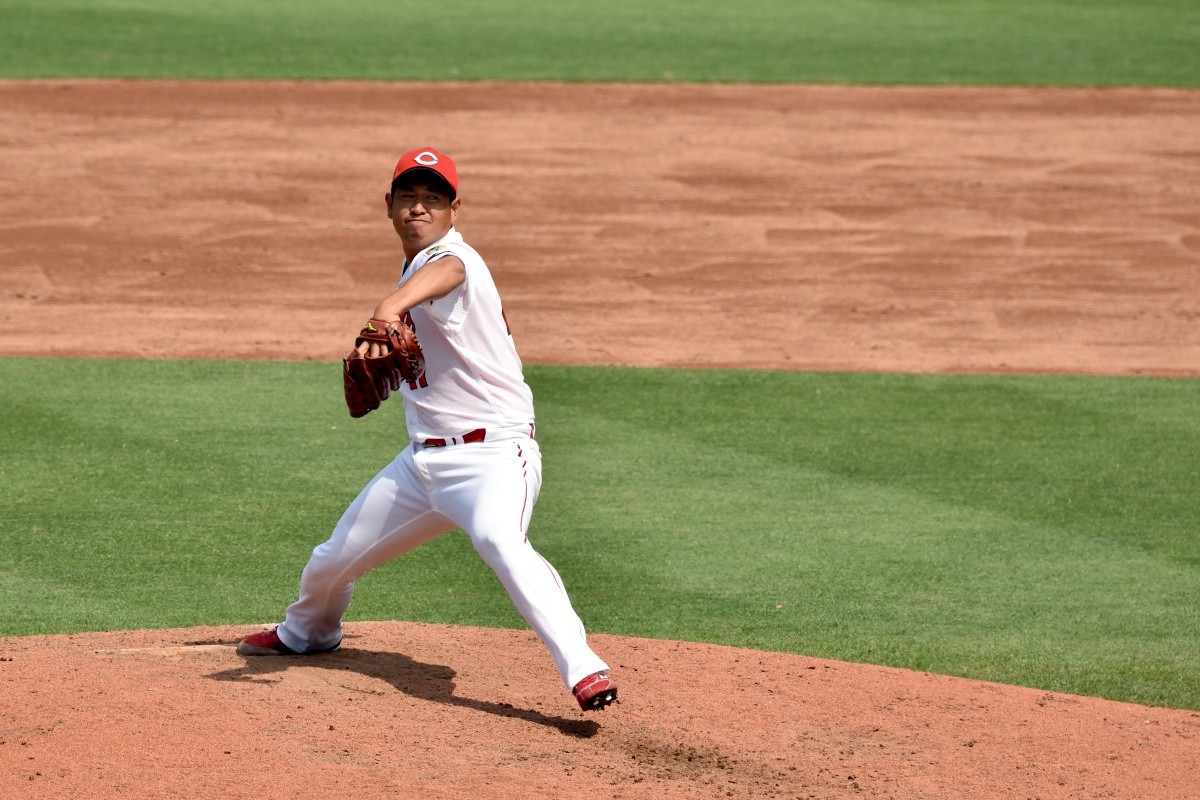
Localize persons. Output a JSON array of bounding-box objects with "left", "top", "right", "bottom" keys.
[{"left": 238, "top": 147, "right": 616, "bottom": 710}]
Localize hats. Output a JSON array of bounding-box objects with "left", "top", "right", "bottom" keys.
[{"left": 391, "top": 147, "right": 458, "bottom": 197}]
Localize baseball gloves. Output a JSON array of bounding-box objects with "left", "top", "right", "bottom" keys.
[{"left": 341, "top": 319, "right": 421, "bottom": 419}]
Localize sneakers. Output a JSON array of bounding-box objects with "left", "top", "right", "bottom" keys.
[
  {"left": 237, "top": 625, "right": 341, "bottom": 656},
  {"left": 572, "top": 673, "right": 617, "bottom": 712}
]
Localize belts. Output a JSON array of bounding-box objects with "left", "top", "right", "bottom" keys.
[{"left": 423, "top": 424, "right": 535, "bottom": 446}]
